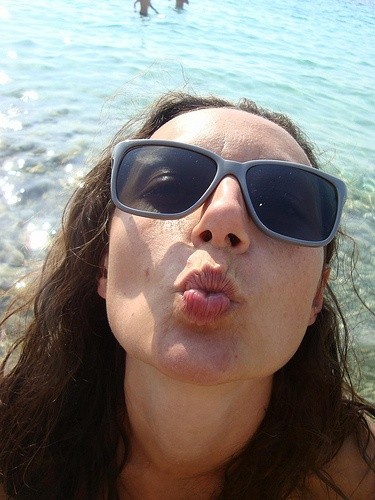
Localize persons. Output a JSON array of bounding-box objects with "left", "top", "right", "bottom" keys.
[
  {"left": 175, "top": 0, "right": 189, "bottom": 11},
  {"left": 0, "top": 88, "right": 375, "bottom": 500},
  {"left": 134, "top": 0, "right": 159, "bottom": 18}
]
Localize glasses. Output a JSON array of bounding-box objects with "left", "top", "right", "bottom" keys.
[{"left": 110, "top": 139, "right": 348, "bottom": 247}]
